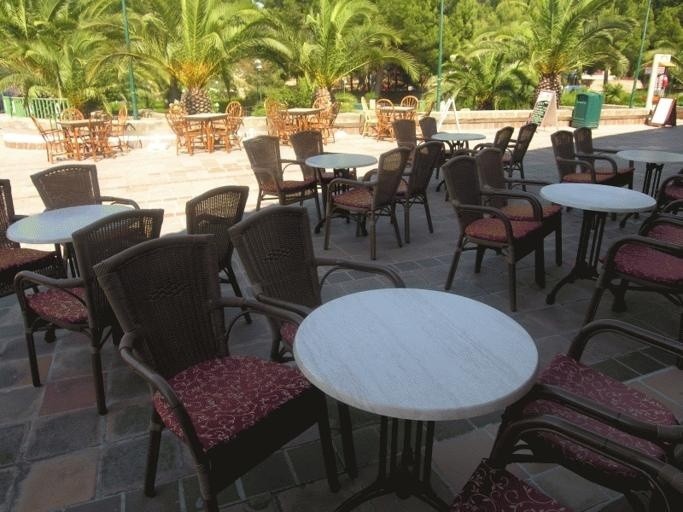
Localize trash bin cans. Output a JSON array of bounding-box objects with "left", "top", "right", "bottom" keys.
[{"left": 569, "top": 92, "right": 603, "bottom": 129}]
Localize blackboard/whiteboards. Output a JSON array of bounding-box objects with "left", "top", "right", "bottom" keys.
[
  {"left": 528, "top": 91, "right": 553, "bottom": 125},
  {"left": 650, "top": 98, "right": 674, "bottom": 124}
]
